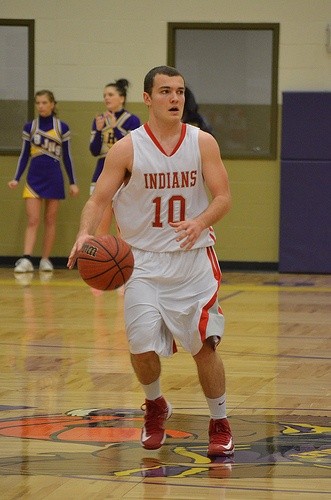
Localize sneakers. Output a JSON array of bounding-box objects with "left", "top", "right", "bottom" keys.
[
  {"left": 40, "top": 259, "right": 52, "bottom": 270},
  {"left": 139, "top": 397, "right": 174, "bottom": 451},
  {"left": 14, "top": 259, "right": 34, "bottom": 272},
  {"left": 207, "top": 419, "right": 233, "bottom": 456}
]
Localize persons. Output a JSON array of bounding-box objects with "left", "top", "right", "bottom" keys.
[
  {"left": 67, "top": 66, "right": 235, "bottom": 455},
  {"left": 89, "top": 79, "right": 141, "bottom": 237},
  {"left": 7, "top": 90, "right": 81, "bottom": 272},
  {"left": 181, "top": 85, "right": 214, "bottom": 137}
]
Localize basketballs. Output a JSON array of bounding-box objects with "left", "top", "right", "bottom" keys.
[{"left": 77, "top": 234, "right": 135, "bottom": 291}]
